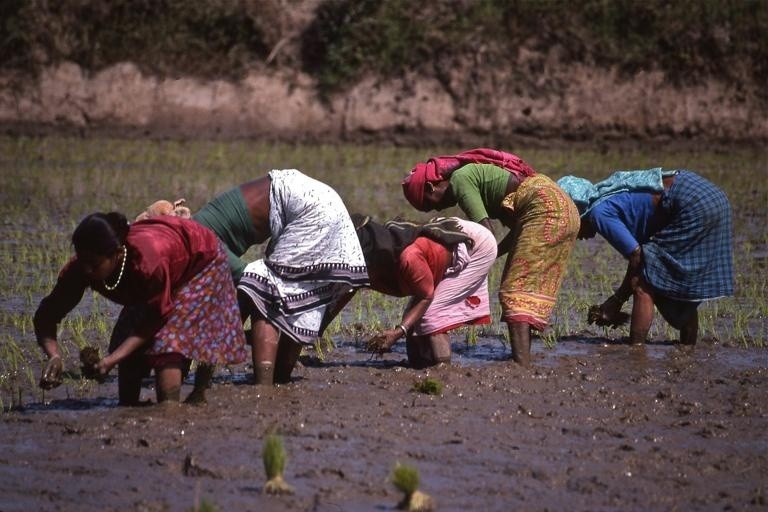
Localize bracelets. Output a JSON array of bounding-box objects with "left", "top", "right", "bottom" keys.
[
  {"left": 395, "top": 325, "right": 408, "bottom": 338},
  {"left": 614, "top": 295, "right": 625, "bottom": 304}
]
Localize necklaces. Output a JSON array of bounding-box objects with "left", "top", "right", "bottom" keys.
[{"left": 102, "top": 244, "right": 128, "bottom": 291}]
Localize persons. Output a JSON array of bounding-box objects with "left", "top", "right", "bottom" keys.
[
  {"left": 135, "top": 169, "right": 370, "bottom": 386},
  {"left": 33, "top": 212, "right": 247, "bottom": 405},
  {"left": 401, "top": 147, "right": 581, "bottom": 367},
  {"left": 318, "top": 213, "right": 499, "bottom": 368},
  {"left": 556, "top": 167, "right": 734, "bottom": 346}
]
